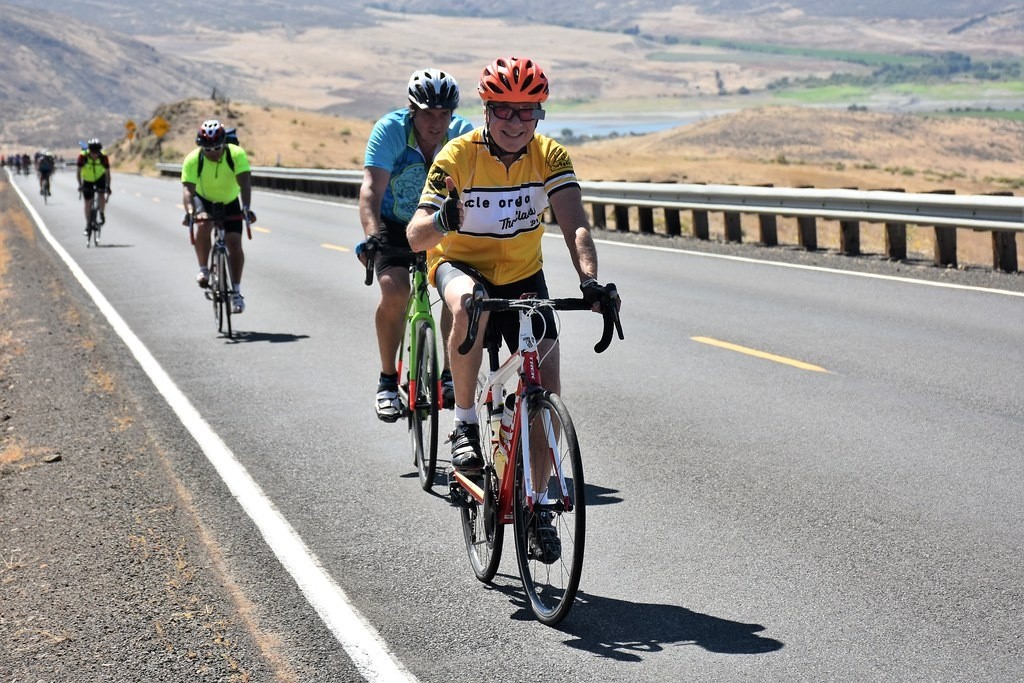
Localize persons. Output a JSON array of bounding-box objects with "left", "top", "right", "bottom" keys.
[
  {"left": 356, "top": 68, "right": 474, "bottom": 423},
  {"left": 180, "top": 120, "right": 257, "bottom": 313},
  {"left": 1, "top": 152, "right": 65, "bottom": 175},
  {"left": 406, "top": 58, "right": 621, "bottom": 564},
  {"left": 36, "top": 156, "right": 54, "bottom": 195},
  {"left": 76, "top": 138, "right": 111, "bottom": 236}
]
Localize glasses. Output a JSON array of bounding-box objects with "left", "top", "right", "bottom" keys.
[
  {"left": 486, "top": 102, "right": 541, "bottom": 122},
  {"left": 201, "top": 143, "right": 223, "bottom": 154}
]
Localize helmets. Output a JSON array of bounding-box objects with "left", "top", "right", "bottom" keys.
[
  {"left": 477, "top": 57, "right": 549, "bottom": 103},
  {"left": 196, "top": 119, "right": 225, "bottom": 147},
  {"left": 88, "top": 139, "right": 102, "bottom": 150},
  {"left": 407, "top": 69, "right": 460, "bottom": 110}
]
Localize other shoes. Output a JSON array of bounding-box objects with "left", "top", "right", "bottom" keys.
[
  {"left": 197, "top": 269, "right": 209, "bottom": 285},
  {"left": 230, "top": 293, "right": 244, "bottom": 314},
  {"left": 84, "top": 227, "right": 89, "bottom": 235},
  {"left": 99, "top": 217, "right": 105, "bottom": 223}
]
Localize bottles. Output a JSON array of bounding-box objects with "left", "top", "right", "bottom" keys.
[
  {"left": 491, "top": 402, "right": 506, "bottom": 479},
  {"left": 499, "top": 392, "right": 518, "bottom": 455}
]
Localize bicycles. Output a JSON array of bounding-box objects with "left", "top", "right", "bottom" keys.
[
  {"left": 78, "top": 184, "right": 110, "bottom": 248},
  {"left": 443, "top": 284, "right": 624, "bottom": 627},
  {"left": 190, "top": 209, "right": 252, "bottom": 338},
  {"left": 37, "top": 172, "right": 52, "bottom": 203},
  {"left": 364, "top": 236, "right": 441, "bottom": 492}
]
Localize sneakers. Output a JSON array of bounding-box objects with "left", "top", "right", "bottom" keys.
[
  {"left": 529, "top": 510, "right": 561, "bottom": 564},
  {"left": 374, "top": 384, "right": 400, "bottom": 422},
  {"left": 441, "top": 369, "right": 456, "bottom": 410},
  {"left": 446, "top": 421, "right": 485, "bottom": 472}
]
